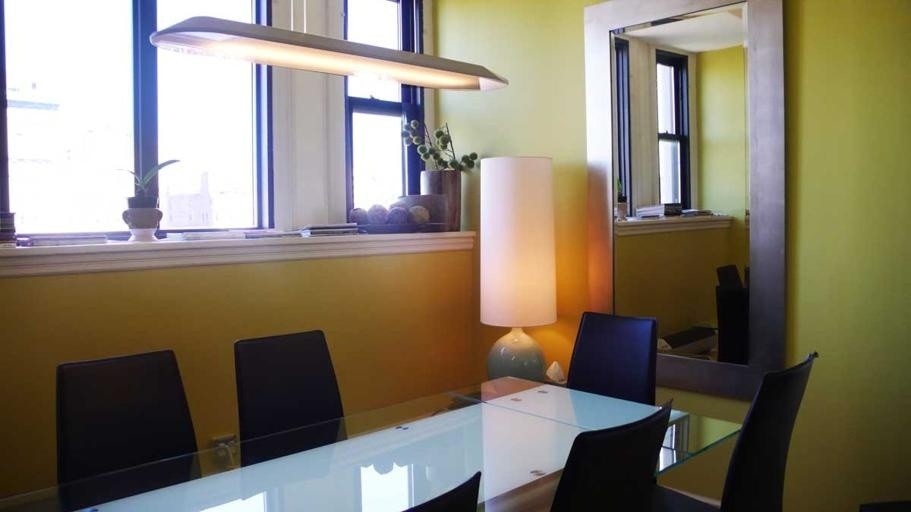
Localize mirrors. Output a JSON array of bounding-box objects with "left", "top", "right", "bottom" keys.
[{"left": 584, "top": 2, "right": 791, "bottom": 404}]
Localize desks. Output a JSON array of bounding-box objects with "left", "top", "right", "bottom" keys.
[{"left": 1, "top": 374, "right": 747, "bottom": 512}]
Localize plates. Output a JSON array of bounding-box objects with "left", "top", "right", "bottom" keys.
[{"left": 358, "top": 222, "right": 425, "bottom": 236}]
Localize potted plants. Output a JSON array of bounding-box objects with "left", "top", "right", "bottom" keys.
[
  {"left": 121, "top": 155, "right": 180, "bottom": 244},
  {"left": 615, "top": 176, "right": 634, "bottom": 222}
]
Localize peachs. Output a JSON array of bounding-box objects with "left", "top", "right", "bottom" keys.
[{"left": 349, "top": 200, "right": 430, "bottom": 224}]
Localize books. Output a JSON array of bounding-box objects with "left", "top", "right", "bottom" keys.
[
  {"left": 164, "top": 221, "right": 360, "bottom": 241},
  {"left": 636, "top": 203, "right": 714, "bottom": 221},
  {"left": 0, "top": 212, "right": 107, "bottom": 249}
]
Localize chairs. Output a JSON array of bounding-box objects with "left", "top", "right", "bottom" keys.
[
  {"left": 549, "top": 396, "right": 676, "bottom": 511},
  {"left": 655, "top": 350, "right": 821, "bottom": 512},
  {"left": 567, "top": 312, "right": 661, "bottom": 407},
  {"left": 55, "top": 348, "right": 202, "bottom": 511},
  {"left": 403, "top": 466, "right": 486, "bottom": 512},
  {"left": 709, "top": 259, "right": 750, "bottom": 367},
  {"left": 233, "top": 325, "right": 349, "bottom": 467}
]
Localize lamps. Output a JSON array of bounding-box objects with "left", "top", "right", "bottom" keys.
[
  {"left": 148, "top": 0, "right": 511, "bottom": 96},
  {"left": 479, "top": 155, "right": 558, "bottom": 382}
]
